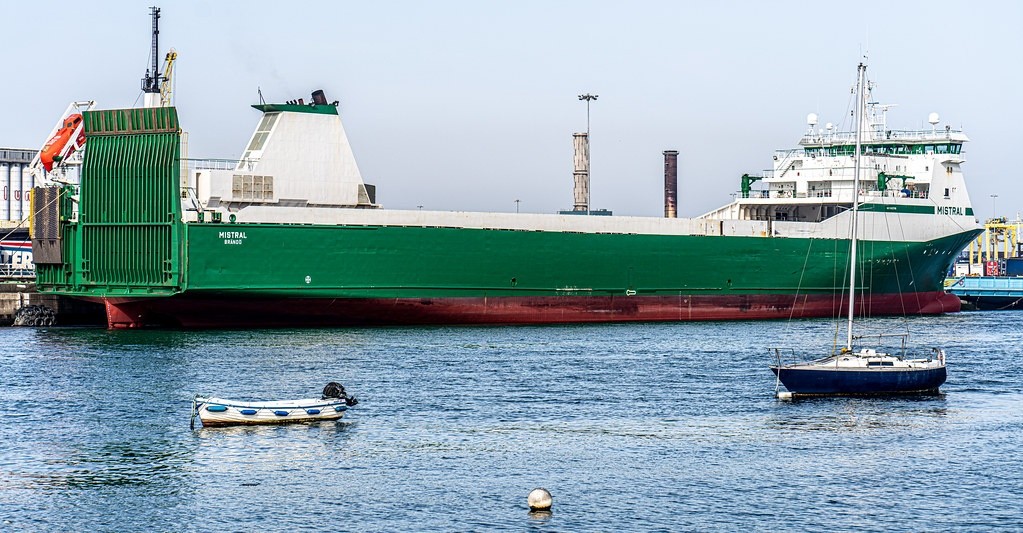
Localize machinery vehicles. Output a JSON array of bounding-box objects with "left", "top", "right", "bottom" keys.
[{"left": 160, "top": 48, "right": 177, "bottom": 107}]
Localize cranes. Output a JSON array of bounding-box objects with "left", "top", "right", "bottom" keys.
[{"left": 182, "top": 132, "right": 189, "bottom": 199}]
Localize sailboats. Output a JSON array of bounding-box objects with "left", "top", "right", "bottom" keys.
[{"left": 766, "top": 63, "right": 947, "bottom": 395}]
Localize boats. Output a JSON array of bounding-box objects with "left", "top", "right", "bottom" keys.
[
  {"left": 195, "top": 382, "right": 359, "bottom": 428},
  {"left": 30, "top": 6, "right": 986, "bottom": 327}
]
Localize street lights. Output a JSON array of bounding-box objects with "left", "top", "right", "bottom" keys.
[
  {"left": 990, "top": 195, "right": 998, "bottom": 276},
  {"left": 515, "top": 200, "right": 519, "bottom": 213},
  {"left": 578, "top": 92, "right": 599, "bottom": 215}
]
